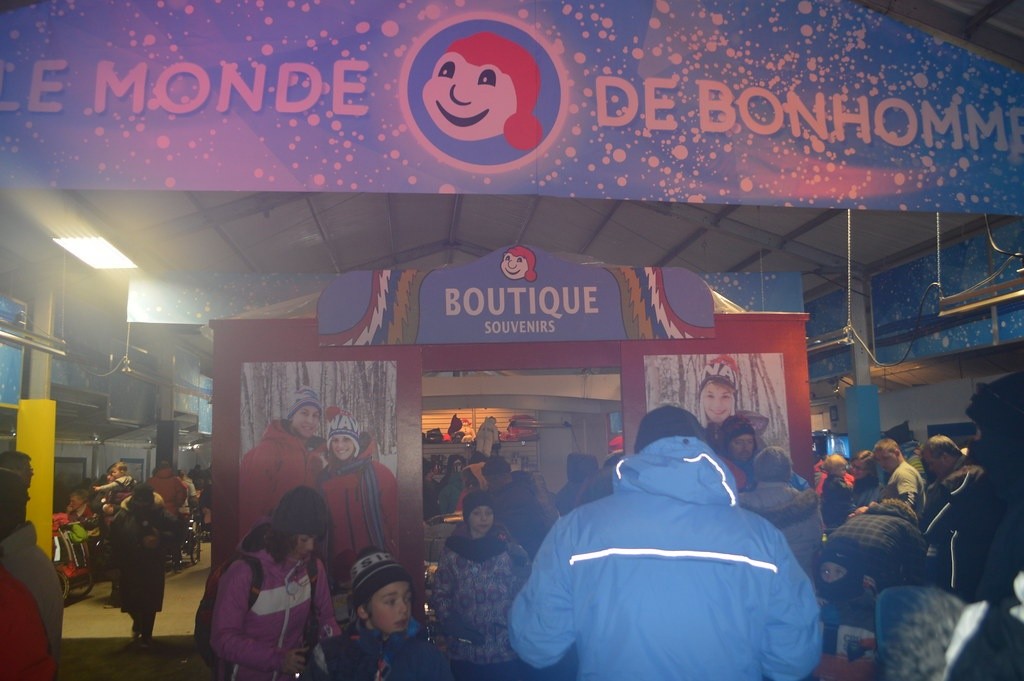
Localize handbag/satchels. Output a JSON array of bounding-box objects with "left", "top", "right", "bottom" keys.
[{"left": 885, "top": 422, "right": 912, "bottom": 445}]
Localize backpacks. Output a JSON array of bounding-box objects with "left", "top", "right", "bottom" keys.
[
  {"left": 109, "top": 510, "right": 146, "bottom": 564},
  {"left": 194, "top": 561, "right": 229, "bottom": 671}
]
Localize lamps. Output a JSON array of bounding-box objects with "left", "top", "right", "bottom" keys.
[{"left": 760, "top": 209, "right": 1024, "bottom": 354}]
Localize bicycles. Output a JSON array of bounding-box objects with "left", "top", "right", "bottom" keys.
[{"left": 171, "top": 505, "right": 202, "bottom": 574}]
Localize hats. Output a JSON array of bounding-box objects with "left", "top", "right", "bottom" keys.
[
  {"left": 718, "top": 415, "right": 755, "bottom": 452},
  {"left": 270, "top": 484, "right": 330, "bottom": 534},
  {"left": 609, "top": 436, "right": 623, "bottom": 453},
  {"left": 699, "top": 356, "right": 739, "bottom": 393},
  {"left": 347, "top": 550, "right": 415, "bottom": 606},
  {"left": 326, "top": 411, "right": 360, "bottom": 458},
  {"left": 481, "top": 457, "right": 511, "bottom": 475},
  {"left": 288, "top": 385, "right": 322, "bottom": 420},
  {"left": 462, "top": 490, "right": 496, "bottom": 518},
  {"left": 634, "top": 404, "right": 712, "bottom": 454}
]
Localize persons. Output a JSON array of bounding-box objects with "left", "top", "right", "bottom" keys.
[
  {"left": 209, "top": 484, "right": 456, "bottom": 681},
  {"left": 507, "top": 373, "right": 1024, "bottom": 681},
  {"left": 421, "top": 453, "right": 542, "bottom": 681},
  {"left": 53, "top": 459, "right": 214, "bottom": 650},
  {"left": 0, "top": 450, "right": 63, "bottom": 681},
  {"left": 696, "top": 356, "right": 766, "bottom": 455},
  {"left": 238, "top": 386, "right": 397, "bottom": 571}
]
